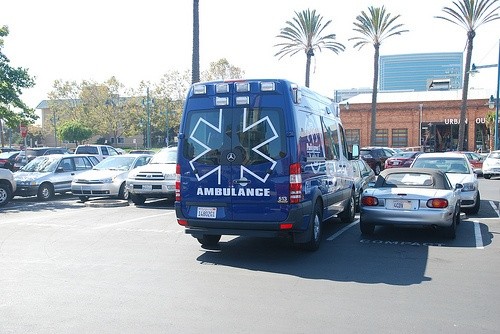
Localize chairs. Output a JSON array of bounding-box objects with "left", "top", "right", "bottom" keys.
[
  {"left": 424, "top": 164, "right": 438, "bottom": 169},
  {"left": 449, "top": 163, "right": 467, "bottom": 171},
  {"left": 423, "top": 179, "right": 433, "bottom": 185},
  {"left": 136, "top": 160, "right": 143, "bottom": 166},
  {"left": 63, "top": 163, "right": 70, "bottom": 170}
]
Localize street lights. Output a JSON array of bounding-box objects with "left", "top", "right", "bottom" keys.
[
  {"left": 49, "top": 111, "right": 60, "bottom": 146},
  {"left": 466, "top": 38, "right": 500, "bottom": 150},
  {"left": 140, "top": 87, "right": 155, "bottom": 149}
]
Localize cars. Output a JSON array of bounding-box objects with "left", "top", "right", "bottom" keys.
[
  {"left": 70, "top": 153, "right": 154, "bottom": 202},
  {"left": 0, "top": 143, "right": 155, "bottom": 202},
  {"left": 358, "top": 146, "right": 500, "bottom": 179},
  {"left": 13, "top": 153, "right": 103, "bottom": 200},
  {"left": 125, "top": 147, "right": 179, "bottom": 207},
  {"left": 348, "top": 160, "right": 375, "bottom": 213},
  {"left": 0, "top": 167, "right": 17, "bottom": 207},
  {"left": 409, "top": 152, "right": 480, "bottom": 215}
]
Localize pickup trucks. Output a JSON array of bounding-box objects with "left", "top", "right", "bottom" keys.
[{"left": 359, "top": 167, "right": 463, "bottom": 240}]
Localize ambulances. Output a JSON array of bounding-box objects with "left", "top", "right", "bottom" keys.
[{"left": 174, "top": 78, "right": 360, "bottom": 254}]
